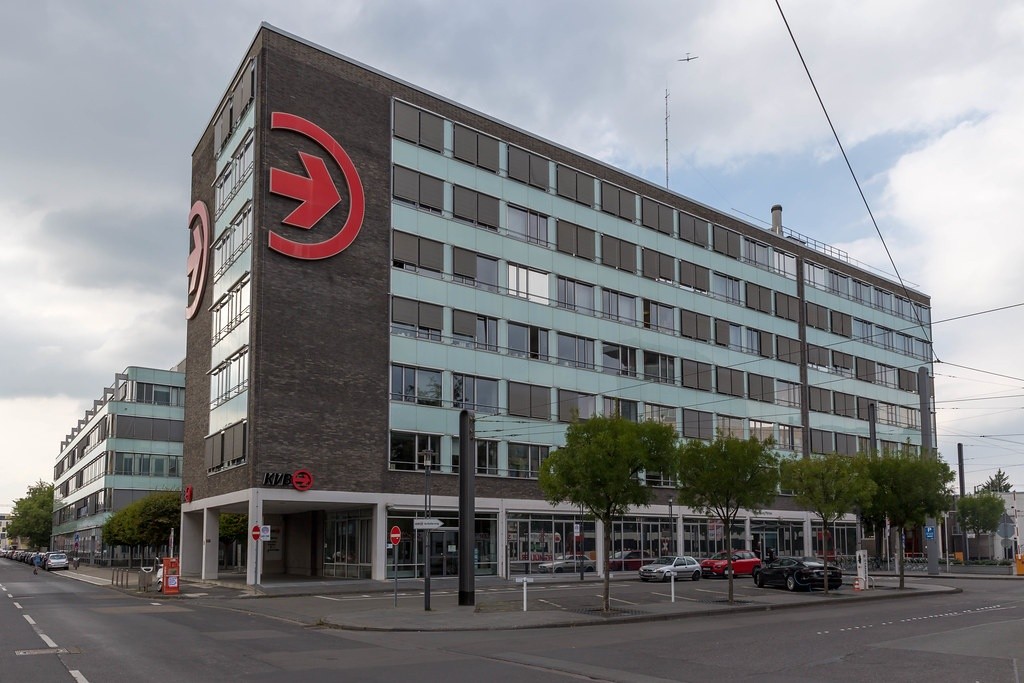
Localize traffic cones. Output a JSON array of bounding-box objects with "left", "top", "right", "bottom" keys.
[{"left": 853, "top": 579, "right": 861, "bottom": 591}]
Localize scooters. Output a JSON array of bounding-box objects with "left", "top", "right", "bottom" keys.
[{"left": 155, "top": 557, "right": 163, "bottom": 592}]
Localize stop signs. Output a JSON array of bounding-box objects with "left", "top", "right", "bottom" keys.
[
  {"left": 251, "top": 525, "right": 261, "bottom": 540},
  {"left": 391, "top": 526, "right": 401, "bottom": 545}
]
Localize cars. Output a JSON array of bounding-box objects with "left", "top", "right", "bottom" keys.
[
  {"left": 639, "top": 556, "right": 702, "bottom": 582},
  {"left": 755, "top": 557, "right": 843, "bottom": 592},
  {"left": 606, "top": 550, "right": 650, "bottom": 572},
  {"left": 0, "top": 548, "right": 69, "bottom": 571},
  {"left": 538, "top": 555, "right": 594, "bottom": 573},
  {"left": 700, "top": 550, "right": 764, "bottom": 580}
]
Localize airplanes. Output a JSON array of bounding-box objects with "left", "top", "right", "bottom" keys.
[{"left": 678, "top": 53, "right": 697, "bottom": 62}]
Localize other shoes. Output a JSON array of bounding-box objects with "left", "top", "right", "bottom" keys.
[
  {"left": 33, "top": 571, "right": 35, "bottom": 574},
  {"left": 35, "top": 572, "right": 38, "bottom": 575}
]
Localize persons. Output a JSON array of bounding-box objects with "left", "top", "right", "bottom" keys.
[{"left": 33, "top": 550, "right": 42, "bottom": 575}]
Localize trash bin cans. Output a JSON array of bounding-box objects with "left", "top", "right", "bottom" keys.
[
  {"left": 73, "top": 557, "right": 79, "bottom": 568},
  {"left": 139, "top": 566, "right": 153, "bottom": 586},
  {"left": 1016, "top": 553, "right": 1024, "bottom": 576}
]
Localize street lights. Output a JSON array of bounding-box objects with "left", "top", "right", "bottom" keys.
[
  {"left": 418, "top": 449, "right": 438, "bottom": 611},
  {"left": 667, "top": 495, "right": 674, "bottom": 556}
]
